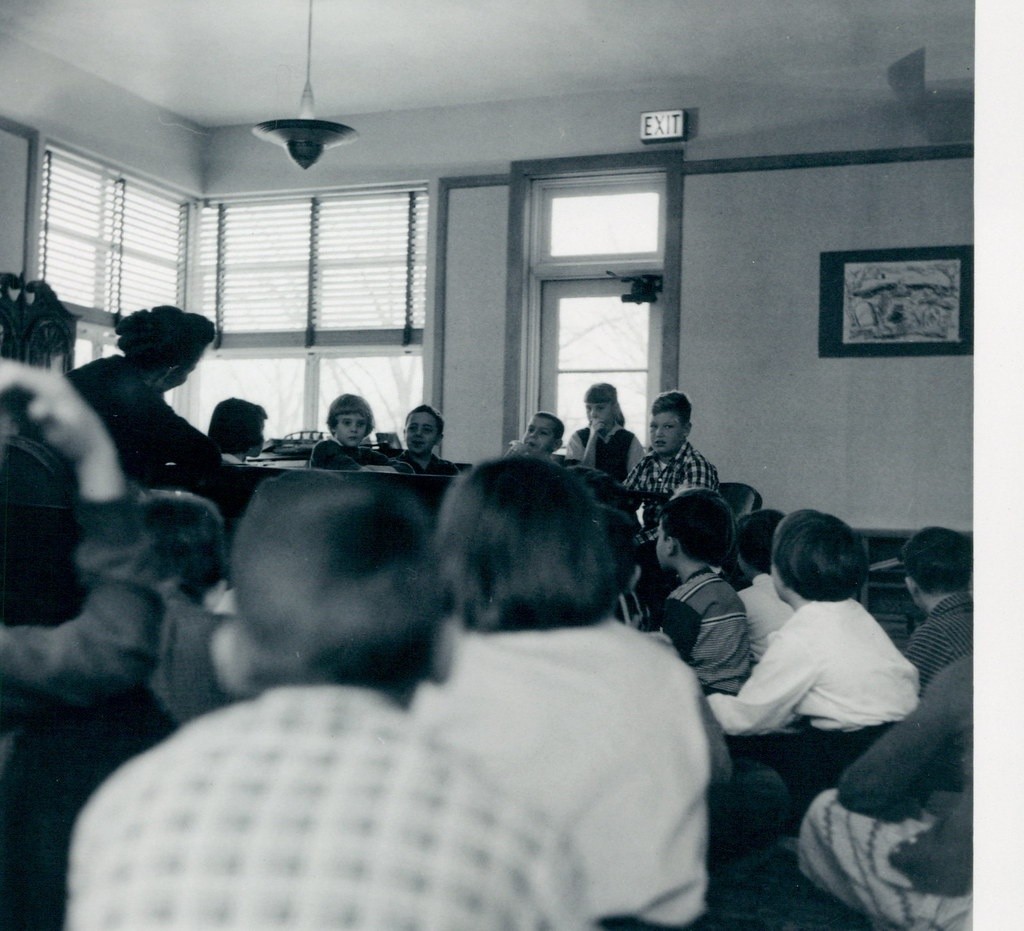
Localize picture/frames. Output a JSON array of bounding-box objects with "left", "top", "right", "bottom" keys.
[{"left": 818, "top": 245, "right": 974, "bottom": 358}]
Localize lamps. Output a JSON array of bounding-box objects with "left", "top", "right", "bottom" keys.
[{"left": 251, "top": 0, "right": 359, "bottom": 169}]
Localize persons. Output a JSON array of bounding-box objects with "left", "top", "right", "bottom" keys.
[
  {"left": 0, "top": 307, "right": 919, "bottom": 931},
  {"left": 391, "top": 405, "right": 459, "bottom": 473},
  {"left": 901, "top": 527, "right": 973, "bottom": 698},
  {"left": 65, "top": 470, "right": 600, "bottom": 931},
  {"left": 797, "top": 655, "right": 973, "bottom": 931},
  {"left": 310, "top": 395, "right": 414, "bottom": 472},
  {"left": 622, "top": 391, "right": 718, "bottom": 579},
  {"left": 505, "top": 412, "right": 564, "bottom": 463},
  {"left": 567, "top": 383, "right": 642, "bottom": 480}
]
